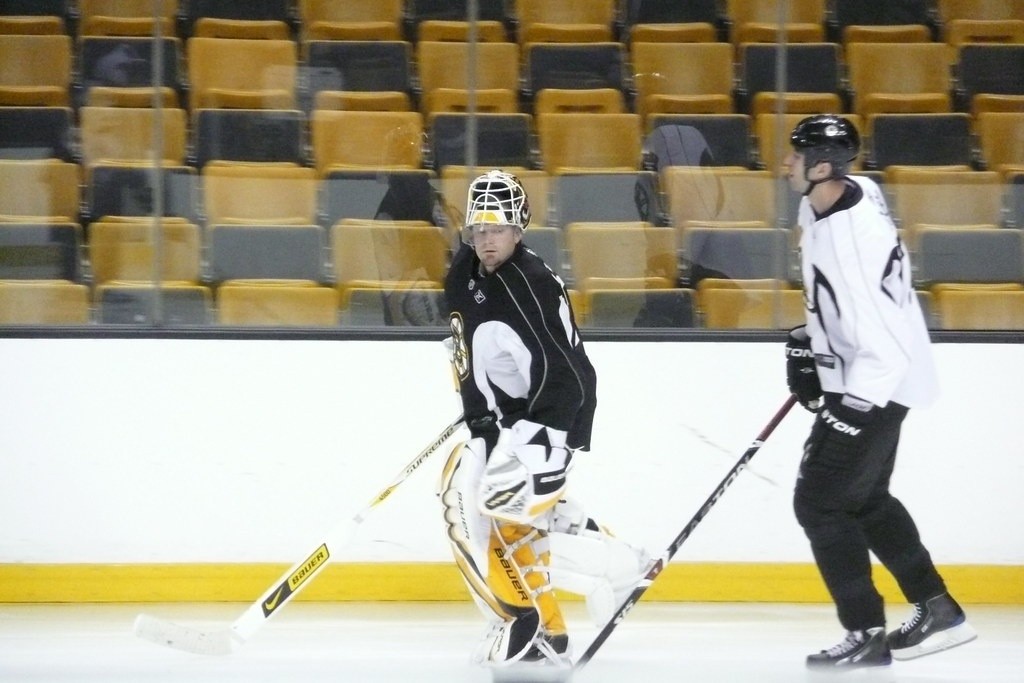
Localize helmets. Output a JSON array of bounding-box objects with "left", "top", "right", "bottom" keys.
[
  {"left": 465, "top": 170, "right": 530, "bottom": 233},
  {"left": 790, "top": 116, "right": 860, "bottom": 179}
]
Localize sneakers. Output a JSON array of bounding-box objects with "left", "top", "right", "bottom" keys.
[
  {"left": 886, "top": 590, "right": 977, "bottom": 661},
  {"left": 807, "top": 627, "right": 890, "bottom": 676}
]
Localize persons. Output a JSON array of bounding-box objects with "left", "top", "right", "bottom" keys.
[
  {"left": 443, "top": 169, "right": 597, "bottom": 682},
  {"left": 635, "top": 125, "right": 752, "bottom": 329},
  {"left": 784, "top": 115, "right": 976, "bottom": 674},
  {"left": 373, "top": 124, "right": 460, "bottom": 325}
]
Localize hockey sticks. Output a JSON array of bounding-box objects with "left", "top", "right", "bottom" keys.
[
  {"left": 133, "top": 412, "right": 466, "bottom": 657},
  {"left": 490, "top": 393, "right": 797, "bottom": 682}
]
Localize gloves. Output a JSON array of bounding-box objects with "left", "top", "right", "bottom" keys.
[
  {"left": 800, "top": 397, "right": 877, "bottom": 485},
  {"left": 787, "top": 323, "right": 825, "bottom": 413}
]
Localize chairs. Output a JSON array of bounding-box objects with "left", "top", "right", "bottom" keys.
[{"left": 0, "top": 0, "right": 1024, "bottom": 329}]
